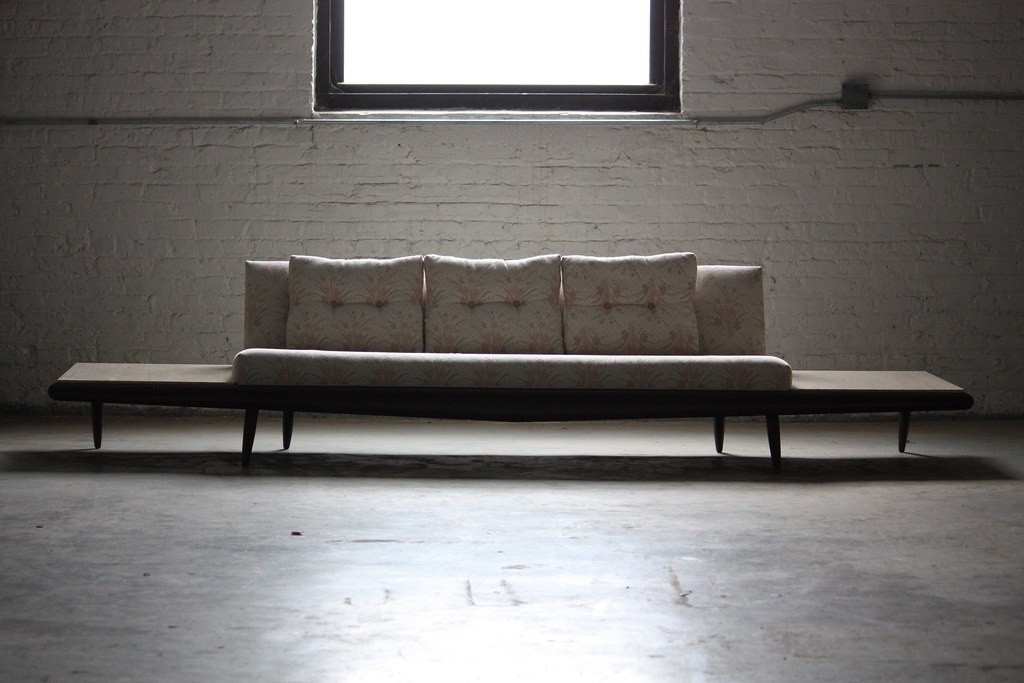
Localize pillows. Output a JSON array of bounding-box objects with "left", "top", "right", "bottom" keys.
[
  {"left": 286, "top": 255, "right": 424, "bottom": 353},
  {"left": 560, "top": 254, "right": 703, "bottom": 355},
  {"left": 424, "top": 256, "right": 564, "bottom": 354}
]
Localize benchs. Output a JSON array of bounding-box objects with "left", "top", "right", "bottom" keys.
[
  {"left": 47, "top": 362, "right": 974, "bottom": 467},
  {"left": 232, "top": 254, "right": 792, "bottom": 467}
]
